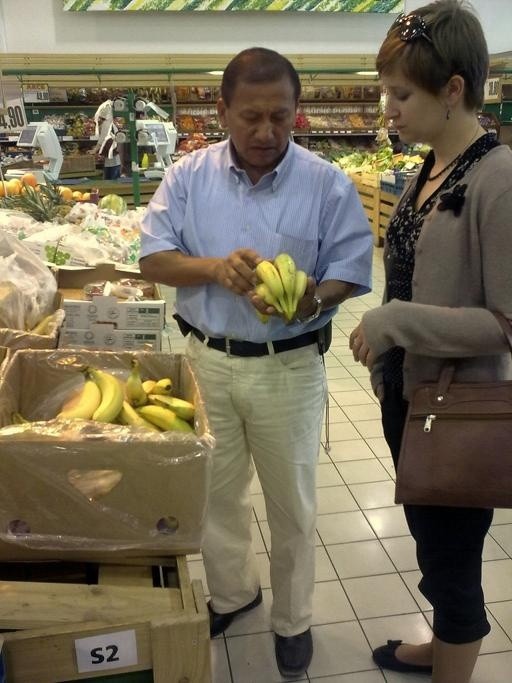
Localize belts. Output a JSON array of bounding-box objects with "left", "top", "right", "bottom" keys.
[{"left": 188, "top": 324, "right": 318, "bottom": 355}]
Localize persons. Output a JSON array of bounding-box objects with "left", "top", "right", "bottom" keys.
[
  {"left": 86, "top": 115, "right": 122, "bottom": 180},
  {"left": 349, "top": 1, "right": 512, "bottom": 683},
  {"left": 137, "top": 48, "right": 374, "bottom": 677}
]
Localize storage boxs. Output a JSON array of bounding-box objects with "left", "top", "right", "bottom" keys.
[
  {"left": 0, "top": 290, "right": 65, "bottom": 356},
  {"left": 0, "top": 345, "right": 11, "bottom": 376},
  {"left": 50, "top": 263, "right": 165, "bottom": 352},
  {"left": 0, "top": 348, "right": 212, "bottom": 564}
]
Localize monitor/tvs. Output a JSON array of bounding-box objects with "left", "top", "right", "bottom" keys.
[
  {"left": 144, "top": 122, "right": 170, "bottom": 146},
  {"left": 16, "top": 126, "right": 38, "bottom": 147}
]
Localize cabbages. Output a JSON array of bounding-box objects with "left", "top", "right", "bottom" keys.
[{"left": 339, "top": 153, "right": 365, "bottom": 168}]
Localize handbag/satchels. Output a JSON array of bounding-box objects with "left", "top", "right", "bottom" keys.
[{"left": 394, "top": 381, "right": 512, "bottom": 508}]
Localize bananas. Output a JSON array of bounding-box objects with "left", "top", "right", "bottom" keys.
[
  {"left": 249, "top": 255, "right": 307, "bottom": 326},
  {"left": 27, "top": 314, "right": 54, "bottom": 335},
  {"left": 9, "top": 358, "right": 195, "bottom": 435}
]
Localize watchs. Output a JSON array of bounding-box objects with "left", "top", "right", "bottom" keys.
[{"left": 296, "top": 296, "right": 323, "bottom": 324}]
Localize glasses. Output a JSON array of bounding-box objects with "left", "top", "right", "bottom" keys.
[{"left": 392, "top": 12, "right": 442, "bottom": 59}]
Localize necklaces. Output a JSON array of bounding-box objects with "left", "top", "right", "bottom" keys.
[{"left": 427, "top": 124, "right": 480, "bottom": 180}]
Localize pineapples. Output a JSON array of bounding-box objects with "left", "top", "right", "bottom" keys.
[{"left": 1, "top": 174, "right": 75, "bottom": 224}]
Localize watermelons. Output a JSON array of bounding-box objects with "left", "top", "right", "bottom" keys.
[{"left": 99, "top": 192, "right": 128, "bottom": 214}]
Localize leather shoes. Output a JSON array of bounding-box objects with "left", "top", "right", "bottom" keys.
[
  {"left": 276, "top": 628, "right": 312, "bottom": 678},
  {"left": 208, "top": 587, "right": 262, "bottom": 636}
]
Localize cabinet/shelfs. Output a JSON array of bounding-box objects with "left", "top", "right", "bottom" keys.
[{"left": 21, "top": 85, "right": 400, "bottom": 153}]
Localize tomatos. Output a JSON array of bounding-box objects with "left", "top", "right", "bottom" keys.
[{"left": 295, "top": 113, "right": 309, "bottom": 129}]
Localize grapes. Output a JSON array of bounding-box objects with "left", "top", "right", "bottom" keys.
[{"left": 45, "top": 246, "right": 70, "bottom": 265}]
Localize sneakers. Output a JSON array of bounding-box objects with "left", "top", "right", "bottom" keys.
[{"left": 373, "top": 639, "right": 435, "bottom": 674}]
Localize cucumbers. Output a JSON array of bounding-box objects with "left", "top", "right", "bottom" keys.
[{"left": 323, "top": 151, "right": 352, "bottom": 163}]
